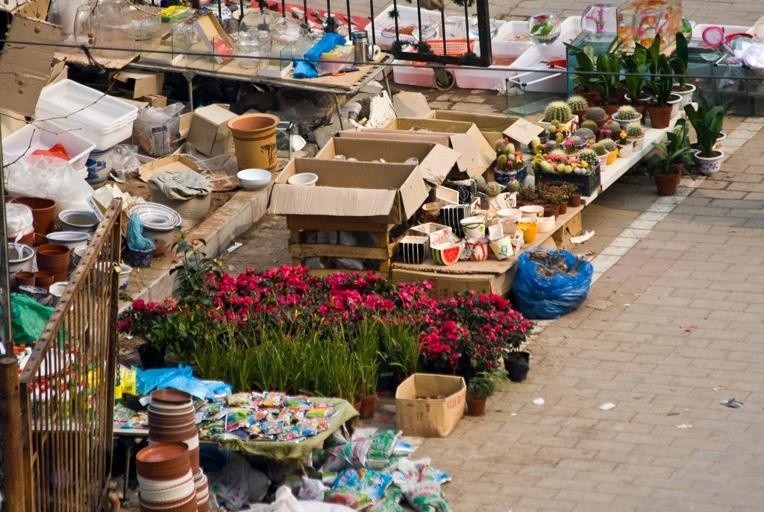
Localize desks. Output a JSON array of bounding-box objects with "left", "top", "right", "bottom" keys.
[
  {"left": 31, "top": 360, "right": 348, "bottom": 504},
  {"left": 55, "top": 30, "right": 395, "bottom": 132}
]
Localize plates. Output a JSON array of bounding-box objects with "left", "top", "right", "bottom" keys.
[
  {"left": 136, "top": 440, "right": 198, "bottom": 512},
  {"left": 147, "top": 390, "right": 209, "bottom": 511}
]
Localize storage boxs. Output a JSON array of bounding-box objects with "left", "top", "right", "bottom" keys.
[
  {"left": 265, "top": 109, "right": 546, "bottom": 228},
  {"left": 394, "top": 372, "right": 467, "bottom": 438}
]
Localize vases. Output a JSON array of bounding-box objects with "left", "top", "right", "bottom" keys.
[
  {"left": 1, "top": 196, "right": 183, "bottom": 341},
  {"left": 428, "top": 350, "right": 530, "bottom": 383},
  {"left": 227, "top": 113, "right": 277, "bottom": 175},
  {"left": 134, "top": 388, "right": 212, "bottom": 511}
]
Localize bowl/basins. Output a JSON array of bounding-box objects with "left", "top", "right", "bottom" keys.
[
  {"left": 236, "top": 168, "right": 272, "bottom": 190},
  {"left": 495, "top": 208, "right": 522, "bottom": 220}
]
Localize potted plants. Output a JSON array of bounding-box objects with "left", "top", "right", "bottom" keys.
[
  {"left": 465, "top": 375, "right": 496, "bottom": 416},
  {"left": 310, "top": 310, "right": 385, "bottom": 420},
  {"left": 492, "top": 30, "right": 727, "bottom": 197}
]
